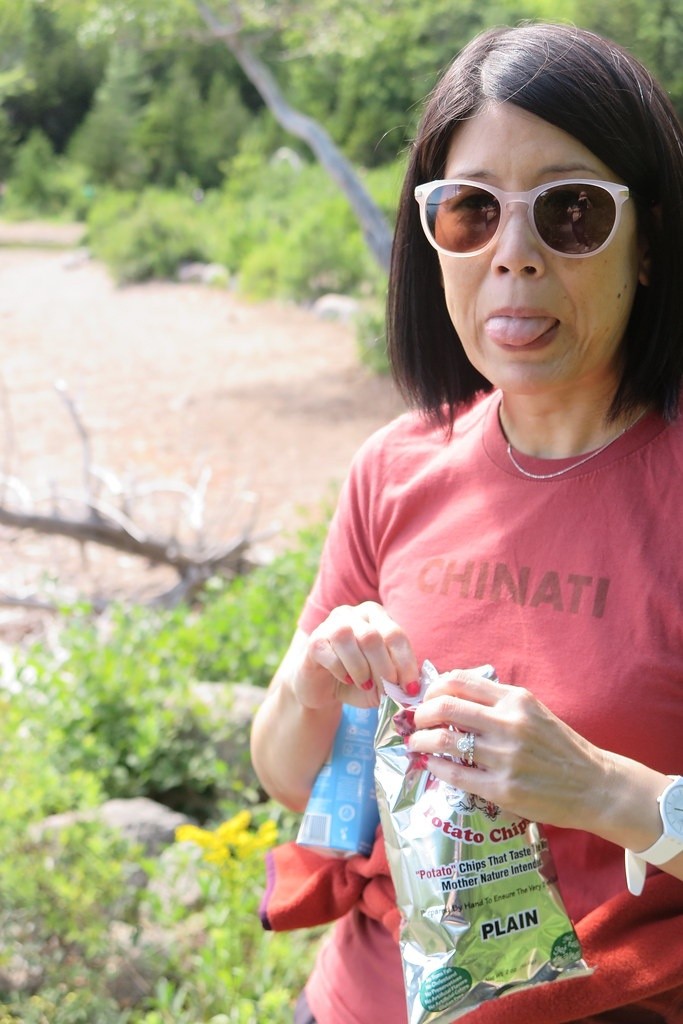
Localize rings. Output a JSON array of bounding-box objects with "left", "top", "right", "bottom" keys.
[{"left": 457, "top": 732, "right": 475, "bottom": 765}]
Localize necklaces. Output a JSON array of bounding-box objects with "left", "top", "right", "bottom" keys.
[{"left": 509, "top": 410, "right": 644, "bottom": 479}]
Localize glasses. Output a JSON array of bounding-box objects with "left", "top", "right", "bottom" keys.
[{"left": 413, "top": 178, "right": 630, "bottom": 258}]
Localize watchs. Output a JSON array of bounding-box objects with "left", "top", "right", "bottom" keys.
[{"left": 625, "top": 775, "right": 682, "bottom": 896}]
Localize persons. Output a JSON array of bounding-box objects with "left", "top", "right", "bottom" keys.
[{"left": 251, "top": 26, "right": 682, "bottom": 1024}]
